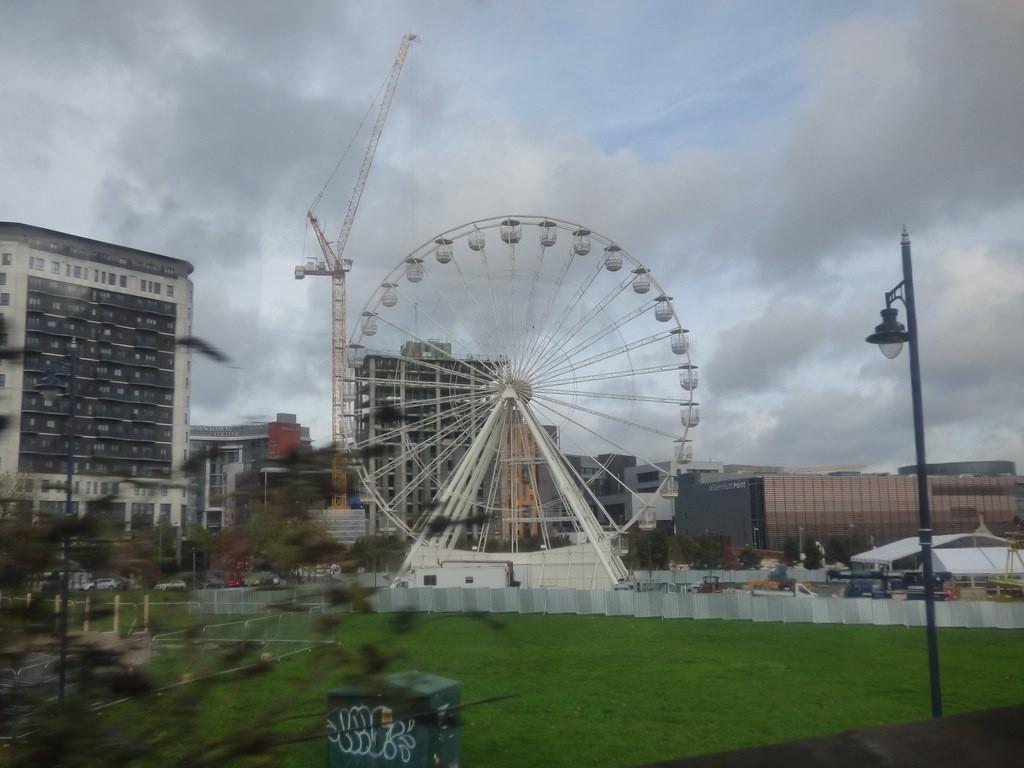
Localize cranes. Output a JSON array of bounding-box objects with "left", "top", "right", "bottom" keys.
[{"left": 294, "top": 31, "right": 422, "bottom": 510}]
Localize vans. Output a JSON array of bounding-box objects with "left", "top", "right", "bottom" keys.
[{"left": 902, "top": 571, "right": 948, "bottom": 601}]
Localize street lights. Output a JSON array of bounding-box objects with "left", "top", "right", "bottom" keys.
[{"left": 864, "top": 222, "right": 944, "bottom": 719}]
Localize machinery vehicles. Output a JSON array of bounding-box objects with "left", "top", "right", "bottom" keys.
[{"left": 696, "top": 574, "right": 723, "bottom": 593}]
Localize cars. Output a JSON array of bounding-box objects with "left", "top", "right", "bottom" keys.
[
  {"left": 81, "top": 578, "right": 117, "bottom": 592},
  {"left": 195, "top": 577, "right": 225, "bottom": 590},
  {"left": 154, "top": 580, "right": 186, "bottom": 591},
  {"left": 223, "top": 575, "right": 245, "bottom": 588}
]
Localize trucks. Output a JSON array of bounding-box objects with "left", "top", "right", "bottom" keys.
[{"left": 751, "top": 583, "right": 818, "bottom": 597}]
